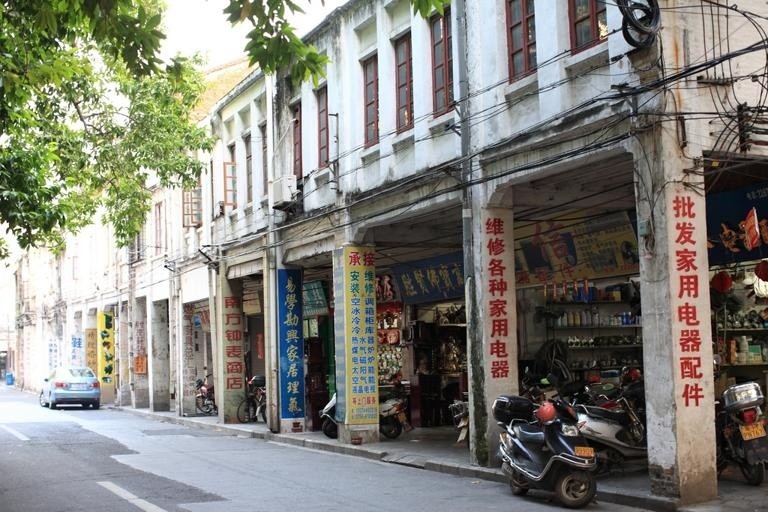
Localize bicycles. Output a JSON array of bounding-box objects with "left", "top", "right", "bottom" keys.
[{"left": 236, "top": 374, "right": 269, "bottom": 425}]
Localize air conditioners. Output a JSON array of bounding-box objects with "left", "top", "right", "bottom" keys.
[{"left": 271, "top": 175, "right": 303, "bottom": 215}]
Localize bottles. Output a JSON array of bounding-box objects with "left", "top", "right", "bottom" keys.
[
  {"left": 739, "top": 335, "right": 749, "bottom": 354},
  {"left": 555, "top": 310, "right": 633, "bottom": 327}
]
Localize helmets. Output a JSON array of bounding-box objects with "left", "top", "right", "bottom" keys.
[
  {"left": 533, "top": 402, "right": 558, "bottom": 423},
  {"left": 629, "top": 368, "right": 642, "bottom": 379}
]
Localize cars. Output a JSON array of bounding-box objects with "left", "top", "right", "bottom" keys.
[{"left": 39, "top": 364, "right": 101, "bottom": 410}]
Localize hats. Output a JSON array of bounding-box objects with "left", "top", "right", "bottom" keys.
[
  {"left": 711, "top": 270, "right": 733, "bottom": 292},
  {"left": 755, "top": 260, "right": 768, "bottom": 281}
]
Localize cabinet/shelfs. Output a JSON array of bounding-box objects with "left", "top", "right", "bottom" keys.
[
  {"left": 710, "top": 256, "right": 768, "bottom": 436},
  {"left": 376, "top": 297, "right": 470, "bottom": 426},
  {"left": 548, "top": 276, "right": 645, "bottom": 396}
]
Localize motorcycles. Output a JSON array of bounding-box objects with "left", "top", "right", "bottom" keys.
[
  {"left": 194, "top": 372, "right": 218, "bottom": 414},
  {"left": 318, "top": 389, "right": 413, "bottom": 439},
  {"left": 448, "top": 366, "right": 648, "bottom": 510},
  {"left": 713, "top": 354, "right": 766, "bottom": 486}
]
